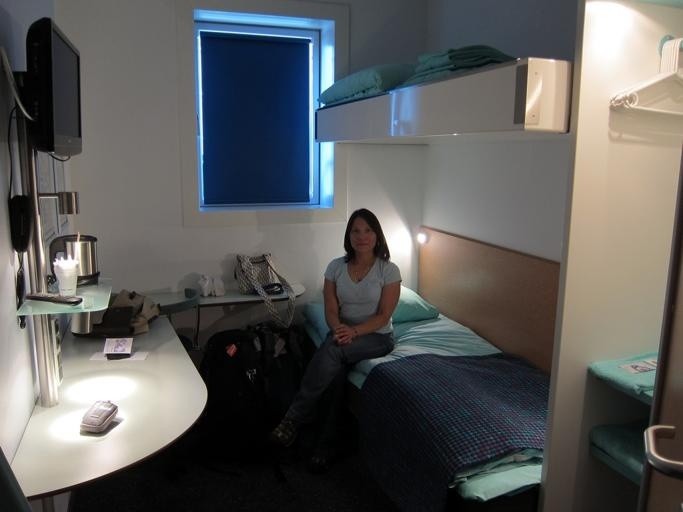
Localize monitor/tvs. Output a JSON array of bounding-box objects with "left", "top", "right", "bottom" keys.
[{"left": 27, "top": 16, "right": 83, "bottom": 157}]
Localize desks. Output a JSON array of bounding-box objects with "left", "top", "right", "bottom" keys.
[{"left": 9, "top": 272, "right": 305, "bottom": 511}]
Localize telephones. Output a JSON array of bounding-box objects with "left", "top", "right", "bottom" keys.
[{"left": 7, "top": 195, "right": 35, "bottom": 253}]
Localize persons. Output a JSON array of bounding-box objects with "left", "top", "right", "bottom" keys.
[{"left": 270, "top": 208, "right": 402, "bottom": 467}]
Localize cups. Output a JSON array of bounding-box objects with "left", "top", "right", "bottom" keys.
[
  {"left": 52, "top": 260, "right": 79, "bottom": 298},
  {"left": 184, "top": 288, "right": 196, "bottom": 298}
]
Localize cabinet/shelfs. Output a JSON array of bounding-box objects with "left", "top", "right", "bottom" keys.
[{"left": 584, "top": 352, "right": 657, "bottom": 487}]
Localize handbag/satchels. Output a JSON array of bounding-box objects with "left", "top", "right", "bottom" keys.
[
  {"left": 235, "top": 253, "right": 276, "bottom": 294},
  {"left": 102, "top": 289, "right": 158, "bottom": 337}
]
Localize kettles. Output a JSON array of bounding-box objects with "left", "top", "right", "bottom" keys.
[{"left": 49, "top": 234, "right": 99, "bottom": 285}]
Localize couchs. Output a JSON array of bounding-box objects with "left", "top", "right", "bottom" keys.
[{"left": 296, "top": 283, "right": 553, "bottom": 509}]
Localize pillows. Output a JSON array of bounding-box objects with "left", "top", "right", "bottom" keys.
[
  {"left": 390, "top": 285, "right": 440, "bottom": 325},
  {"left": 315, "top": 57, "right": 413, "bottom": 106}
]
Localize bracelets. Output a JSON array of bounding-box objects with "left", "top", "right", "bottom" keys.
[{"left": 352, "top": 326, "right": 359, "bottom": 339}]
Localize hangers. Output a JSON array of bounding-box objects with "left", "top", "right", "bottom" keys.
[{"left": 605, "top": 35, "right": 682, "bottom": 118}]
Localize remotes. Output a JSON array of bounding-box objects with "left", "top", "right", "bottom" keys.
[{"left": 25, "top": 292, "right": 83, "bottom": 305}]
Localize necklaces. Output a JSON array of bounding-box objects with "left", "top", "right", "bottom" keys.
[{"left": 349, "top": 267, "right": 367, "bottom": 282}]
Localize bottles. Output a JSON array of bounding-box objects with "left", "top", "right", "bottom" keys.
[{"left": 72, "top": 312, "right": 93, "bottom": 337}]
